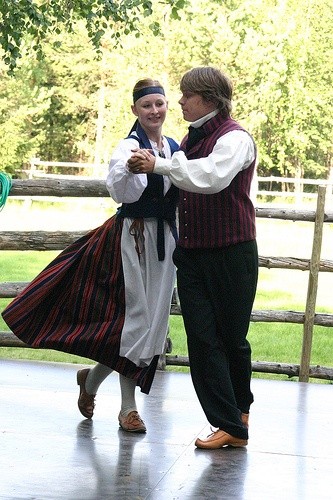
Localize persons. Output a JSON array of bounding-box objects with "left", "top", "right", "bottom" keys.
[
  {"left": 2, "top": 77, "right": 181, "bottom": 433},
  {"left": 130, "top": 66, "right": 259, "bottom": 450}
]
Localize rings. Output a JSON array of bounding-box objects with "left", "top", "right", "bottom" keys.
[{"left": 136, "top": 167, "right": 139, "bottom": 171}]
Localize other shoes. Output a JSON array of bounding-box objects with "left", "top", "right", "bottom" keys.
[
  {"left": 117, "top": 410, "right": 148, "bottom": 433},
  {"left": 77, "top": 368, "right": 97, "bottom": 418}
]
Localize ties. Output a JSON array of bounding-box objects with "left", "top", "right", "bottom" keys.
[{"left": 185, "top": 117, "right": 212, "bottom": 152}]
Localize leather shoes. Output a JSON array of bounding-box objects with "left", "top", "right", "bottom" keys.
[
  {"left": 194, "top": 426, "right": 249, "bottom": 448},
  {"left": 241, "top": 411, "right": 249, "bottom": 428}
]
